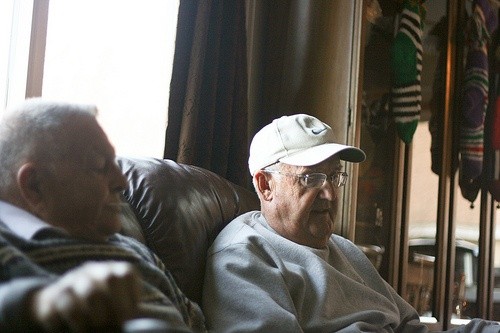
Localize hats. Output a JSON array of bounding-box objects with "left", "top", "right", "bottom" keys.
[{"left": 248, "top": 114, "right": 367, "bottom": 177}]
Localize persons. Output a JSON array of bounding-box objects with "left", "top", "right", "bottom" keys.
[
  {"left": 0, "top": 96, "right": 208, "bottom": 333},
  {"left": 202, "top": 113, "right": 498, "bottom": 333}
]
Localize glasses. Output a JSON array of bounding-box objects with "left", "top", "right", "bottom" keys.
[{"left": 263, "top": 171, "right": 349, "bottom": 189}]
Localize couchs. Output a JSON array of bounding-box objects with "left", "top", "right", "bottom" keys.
[{"left": 113, "top": 155, "right": 261, "bottom": 313}]
